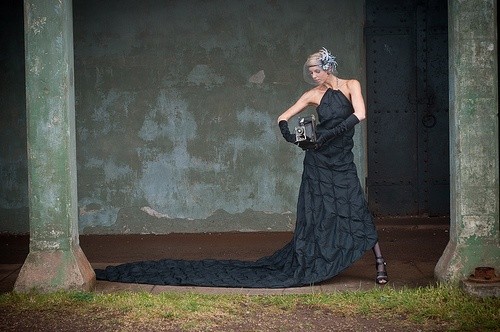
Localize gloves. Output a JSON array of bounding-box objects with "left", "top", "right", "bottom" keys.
[
  {"left": 315, "top": 113, "right": 360, "bottom": 150},
  {"left": 279, "top": 120, "right": 315, "bottom": 151}
]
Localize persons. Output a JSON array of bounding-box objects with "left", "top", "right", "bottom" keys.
[{"left": 277, "top": 46, "right": 389, "bottom": 284}]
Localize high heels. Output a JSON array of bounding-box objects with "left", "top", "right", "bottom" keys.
[{"left": 377, "top": 262, "right": 388, "bottom": 284}]
